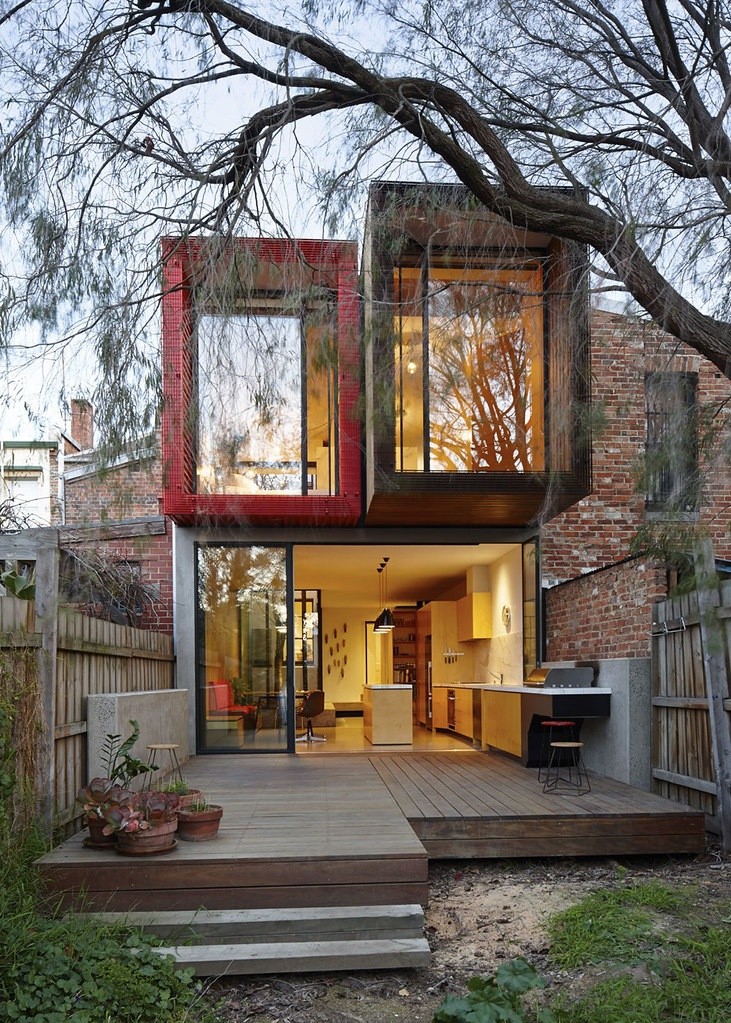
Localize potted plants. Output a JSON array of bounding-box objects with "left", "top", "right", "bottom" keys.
[{"left": 77, "top": 719, "right": 224, "bottom": 856}]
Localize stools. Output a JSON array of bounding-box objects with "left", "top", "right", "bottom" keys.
[
  {"left": 542, "top": 742, "right": 592, "bottom": 796},
  {"left": 141, "top": 745, "right": 183, "bottom": 793},
  {"left": 538, "top": 721, "right": 582, "bottom": 787}
]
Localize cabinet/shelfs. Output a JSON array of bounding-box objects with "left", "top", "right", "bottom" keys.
[
  {"left": 416, "top": 601, "right": 475, "bottom": 730},
  {"left": 434, "top": 687, "right": 481, "bottom": 745},
  {"left": 392, "top": 611, "right": 417, "bottom": 713},
  {"left": 480, "top": 691, "right": 612, "bottom": 768},
  {"left": 363, "top": 684, "right": 413, "bottom": 745}
]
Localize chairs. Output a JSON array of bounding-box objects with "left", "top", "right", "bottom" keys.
[{"left": 295, "top": 691, "right": 326, "bottom": 743}]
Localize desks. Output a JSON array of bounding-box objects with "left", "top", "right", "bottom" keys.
[{"left": 254, "top": 694, "right": 313, "bottom": 743}]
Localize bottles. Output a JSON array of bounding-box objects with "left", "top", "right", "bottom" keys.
[
  {"left": 394, "top": 646, "right": 398, "bottom": 656},
  {"left": 393, "top": 662, "right": 416, "bottom": 683}
]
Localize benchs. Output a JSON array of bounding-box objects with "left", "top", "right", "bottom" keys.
[
  {"left": 206, "top": 680, "right": 257, "bottom": 746},
  {"left": 258, "top": 702, "right": 336, "bottom": 727}
]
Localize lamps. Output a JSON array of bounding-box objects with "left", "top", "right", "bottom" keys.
[
  {"left": 373, "top": 557, "right": 395, "bottom": 633},
  {"left": 407, "top": 339, "right": 417, "bottom": 374}
]
